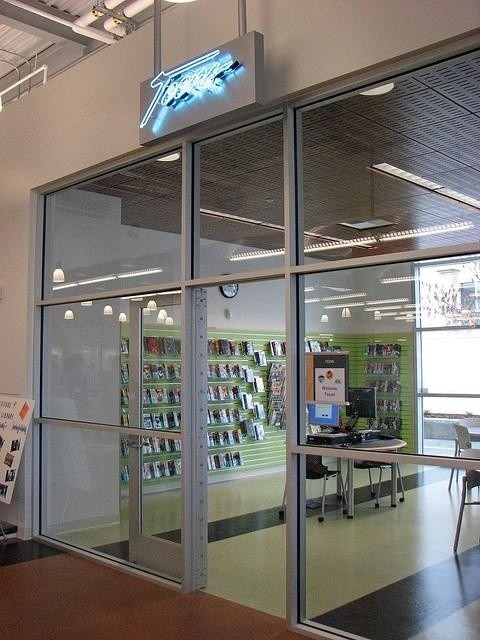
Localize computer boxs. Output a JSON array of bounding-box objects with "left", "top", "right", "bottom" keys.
[
  {"left": 307, "top": 432, "right": 348, "bottom": 445},
  {"left": 346, "top": 429, "right": 381, "bottom": 440}
]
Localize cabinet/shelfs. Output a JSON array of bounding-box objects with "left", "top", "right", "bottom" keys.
[
  {"left": 244, "top": 340, "right": 269, "bottom": 444},
  {"left": 363, "top": 341, "right": 405, "bottom": 438},
  {"left": 120, "top": 331, "right": 246, "bottom": 485},
  {"left": 268, "top": 357, "right": 290, "bottom": 433}
]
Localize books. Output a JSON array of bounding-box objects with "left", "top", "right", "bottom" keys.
[{"left": 122, "top": 337, "right": 402, "bottom": 485}]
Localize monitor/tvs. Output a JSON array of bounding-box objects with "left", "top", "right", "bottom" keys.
[
  {"left": 346, "top": 387, "right": 377, "bottom": 419},
  {"left": 307, "top": 402, "right": 339, "bottom": 427}
]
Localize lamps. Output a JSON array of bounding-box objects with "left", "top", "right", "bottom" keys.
[
  {"left": 313, "top": 305, "right": 420, "bottom": 327},
  {"left": 50, "top": 256, "right": 176, "bottom": 327}
]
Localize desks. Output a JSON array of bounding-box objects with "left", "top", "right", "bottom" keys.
[{"left": 312, "top": 431, "right": 408, "bottom": 520}]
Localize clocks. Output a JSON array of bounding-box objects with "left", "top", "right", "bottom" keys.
[{"left": 218, "top": 273, "right": 239, "bottom": 299}]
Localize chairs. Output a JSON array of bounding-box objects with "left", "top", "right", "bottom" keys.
[
  {"left": 353, "top": 452, "right": 406, "bottom": 508},
  {"left": 454, "top": 444, "right": 480, "bottom": 558},
  {"left": 449, "top": 418, "right": 475, "bottom": 492},
  {"left": 280, "top": 449, "right": 350, "bottom": 524}
]
